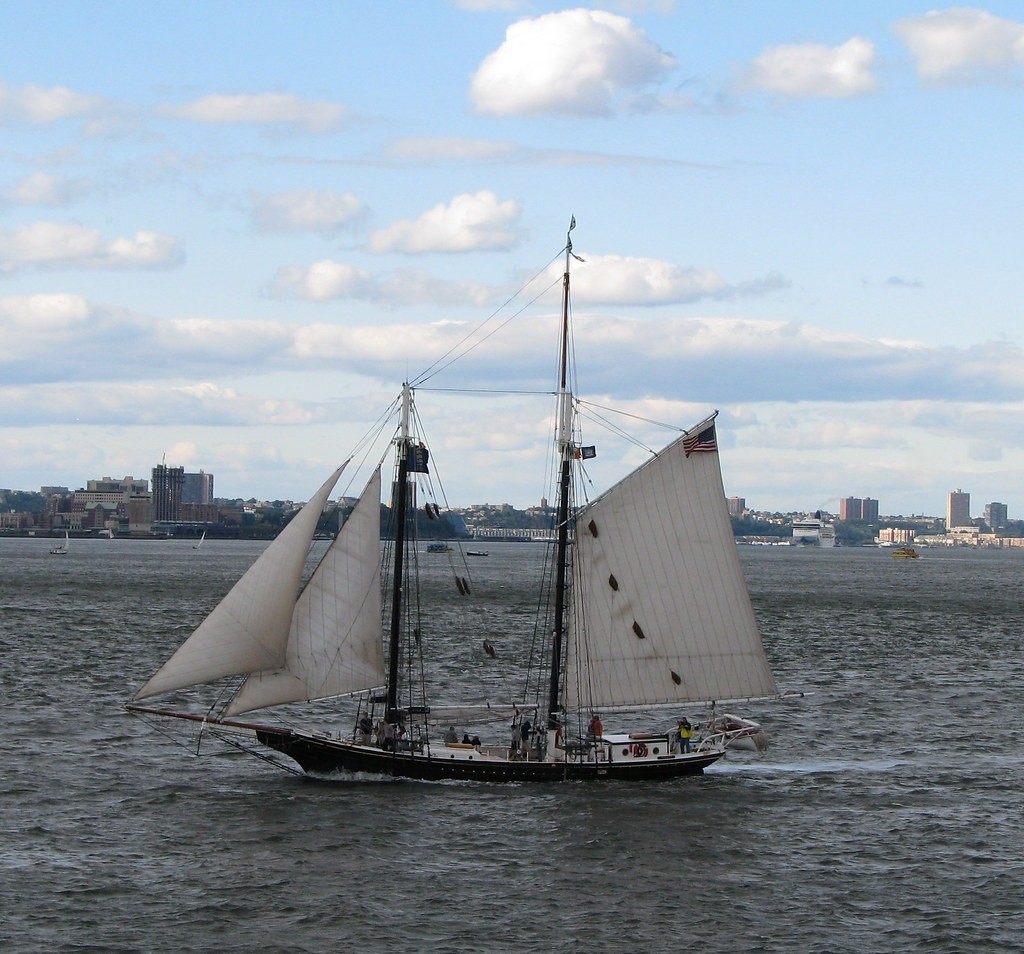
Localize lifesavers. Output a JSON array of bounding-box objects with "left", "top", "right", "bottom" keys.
[{"left": 634, "top": 743, "right": 649, "bottom": 757}]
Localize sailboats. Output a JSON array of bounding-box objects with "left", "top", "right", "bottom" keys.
[
  {"left": 120, "top": 214, "right": 817, "bottom": 786},
  {"left": 192, "top": 531, "right": 206, "bottom": 549},
  {"left": 50, "top": 530, "right": 70, "bottom": 554}
]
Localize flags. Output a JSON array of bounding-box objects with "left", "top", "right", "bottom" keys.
[{"left": 683, "top": 425, "right": 716, "bottom": 458}]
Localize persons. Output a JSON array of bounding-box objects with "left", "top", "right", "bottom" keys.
[
  {"left": 446, "top": 727, "right": 458, "bottom": 743},
  {"left": 359, "top": 711, "right": 406, "bottom": 752},
  {"left": 586, "top": 716, "right": 602, "bottom": 736},
  {"left": 510, "top": 720, "right": 531, "bottom": 749},
  {"left": 462, "top": 734, "right": 471, "bottom": 743},
  {"left": 677, "top": 717, "right": 692, "bottom": 754},
  {"left": 471, "top": 736, "right": 481, "bottom": 746}
]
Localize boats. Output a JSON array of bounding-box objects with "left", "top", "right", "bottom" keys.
[
  {"left": 426, "top": 543, "right": 455, "bottom": 553},
  {"left": 891, "top": 548, "right": 920, "bottom": 559},
  {"left": 466, "top": 550, "right": 489, "bottom": 556}
]
